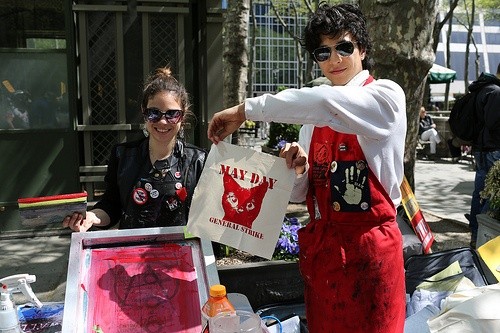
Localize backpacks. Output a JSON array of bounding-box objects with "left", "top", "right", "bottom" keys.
[{"left": 449, "top": 87, "right": 499, "bottom": 137}]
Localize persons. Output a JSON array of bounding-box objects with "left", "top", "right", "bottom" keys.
[
  {"left": 207, "top": 5, "right": 407, "bottom": 333},
  {"left": 469, "top": 64, "right": 500, "bottom": 215},
  {"left": 62, "top": 66, "right": 209, "bottom": 233},
  {"left": 418, "top": 107, "right": 443, "bottom": 161}
]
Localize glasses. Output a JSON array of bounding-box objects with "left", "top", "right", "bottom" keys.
[
  {"left": 312, "top": 40, "right": 359, "bottom": 61},
  {"left": 143, "top": 107, "right": 184, "bottom": 124}
]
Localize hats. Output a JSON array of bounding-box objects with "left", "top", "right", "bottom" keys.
[{"left": 306, "top": 76, "right": 334, "bottom": 88}]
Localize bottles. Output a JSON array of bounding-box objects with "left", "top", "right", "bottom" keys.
[{"left": 201, "top": 284, "right": 236, "bottom": 333}]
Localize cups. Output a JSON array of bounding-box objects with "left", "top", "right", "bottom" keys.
[{"left": 209, "top": 310, "right": 262, "bottom": 333}]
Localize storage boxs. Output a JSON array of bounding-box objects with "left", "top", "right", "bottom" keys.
[{"left": 476, "top": 235, "right": 500, "bottom": 283}]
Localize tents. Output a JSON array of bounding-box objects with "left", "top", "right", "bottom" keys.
[{"left": 426, "top": 63, "right": 457, "bottom": 111}]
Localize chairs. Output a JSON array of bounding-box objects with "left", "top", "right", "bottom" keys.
[{"left": 417, "top": 135, "right": 438, "bottom": 160}]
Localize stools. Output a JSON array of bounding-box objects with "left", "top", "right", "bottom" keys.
[{"left": 455, "top": 147, "right": 475, "bottom": 166}]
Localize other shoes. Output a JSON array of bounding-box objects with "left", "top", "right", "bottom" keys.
[
  {"left": 438, "top": 143, "right": 447, "bottom": 148},
  {"left": 469, "top": 235, "right": 477, "bottom": 249},
  {"left": 430, "top": 154, "right": 440, "bottom": 159}
]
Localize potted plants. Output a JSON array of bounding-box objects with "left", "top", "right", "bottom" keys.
[{"left": 475, "top": 159, "right": 500, "bottom": 250}]
